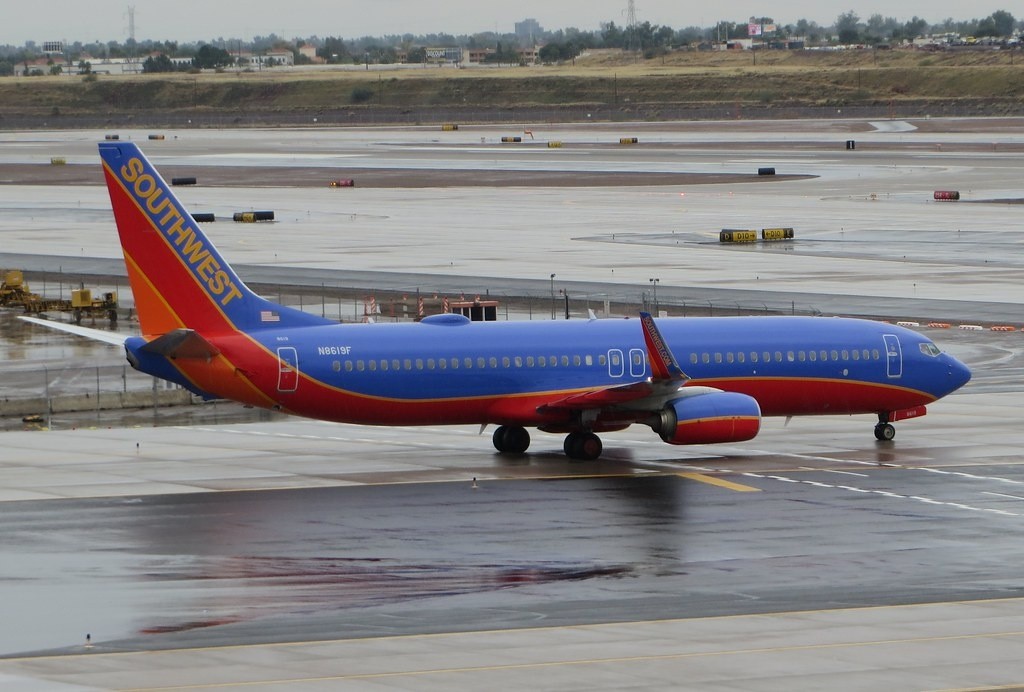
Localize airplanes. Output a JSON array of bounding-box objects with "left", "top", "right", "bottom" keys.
[{"left": 17, "top": 141, "right": 973, "bottom": 463}]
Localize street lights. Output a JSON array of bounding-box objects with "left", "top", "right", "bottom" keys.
[
  {"left": 649, "top": 278, "right": 660, "bottom": 318},
  {"left": 550, "top": 273, "right": 557, "bottom": 320}
]
